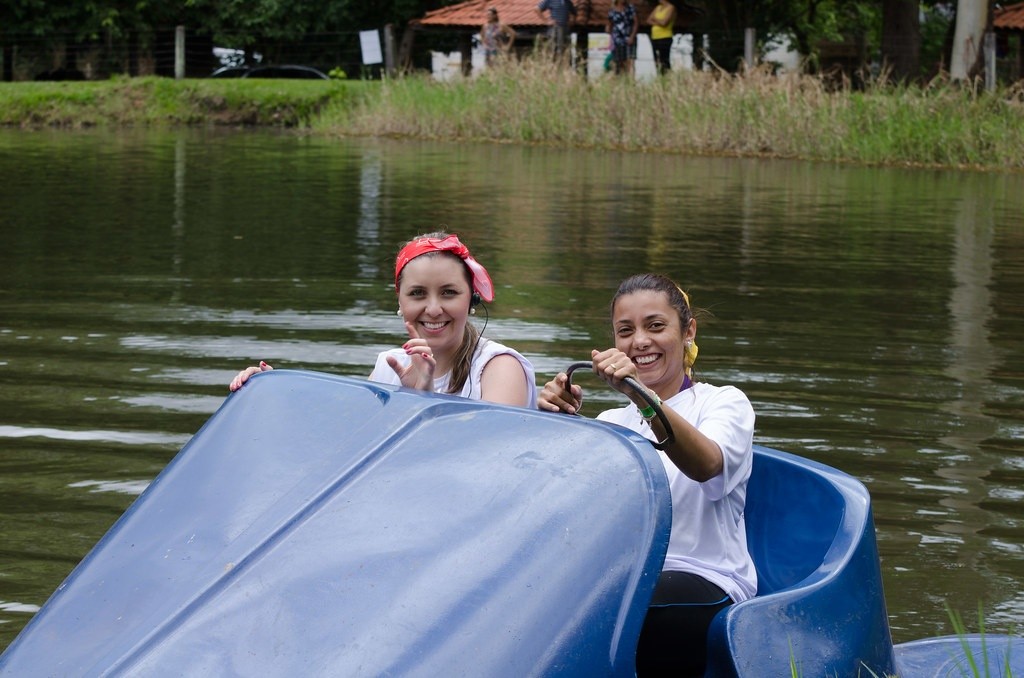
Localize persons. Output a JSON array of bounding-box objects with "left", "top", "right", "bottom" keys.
[
  {"left": 604, "top": 0, "right": 639, "bottom": 74},
  {"left": 534, "top": 0, "right": 578, "bottom": 51},
  {"left": 230, "top": 232, "right": 538, "bottom": 409},
  {"left": 646, "top": 0, "right": 677, "bottom": 76},
  {"left": 480, "top": 8, "right": 516, "bottom": 62},
  {"left": 536, "top": 274, "right": 757, "bottom": 678}
]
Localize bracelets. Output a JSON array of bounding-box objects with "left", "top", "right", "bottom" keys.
[{"left": 636, "top": 390, "right": 663, "bottom": 428}]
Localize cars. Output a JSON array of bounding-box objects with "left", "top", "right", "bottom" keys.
[{"left": 211, "top": 63, "right": 333, "bottom": 81}]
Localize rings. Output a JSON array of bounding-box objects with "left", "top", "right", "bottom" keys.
[
  {"left": 611, "top": 364, "right": 616, "bottom": 372},
  {"left": 431, "top": 354, "right": 433, "bottom": 357}
]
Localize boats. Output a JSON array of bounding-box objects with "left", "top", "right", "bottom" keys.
[{"left": 0, "top": 361, "right": 1024, "bottom": 678}]
